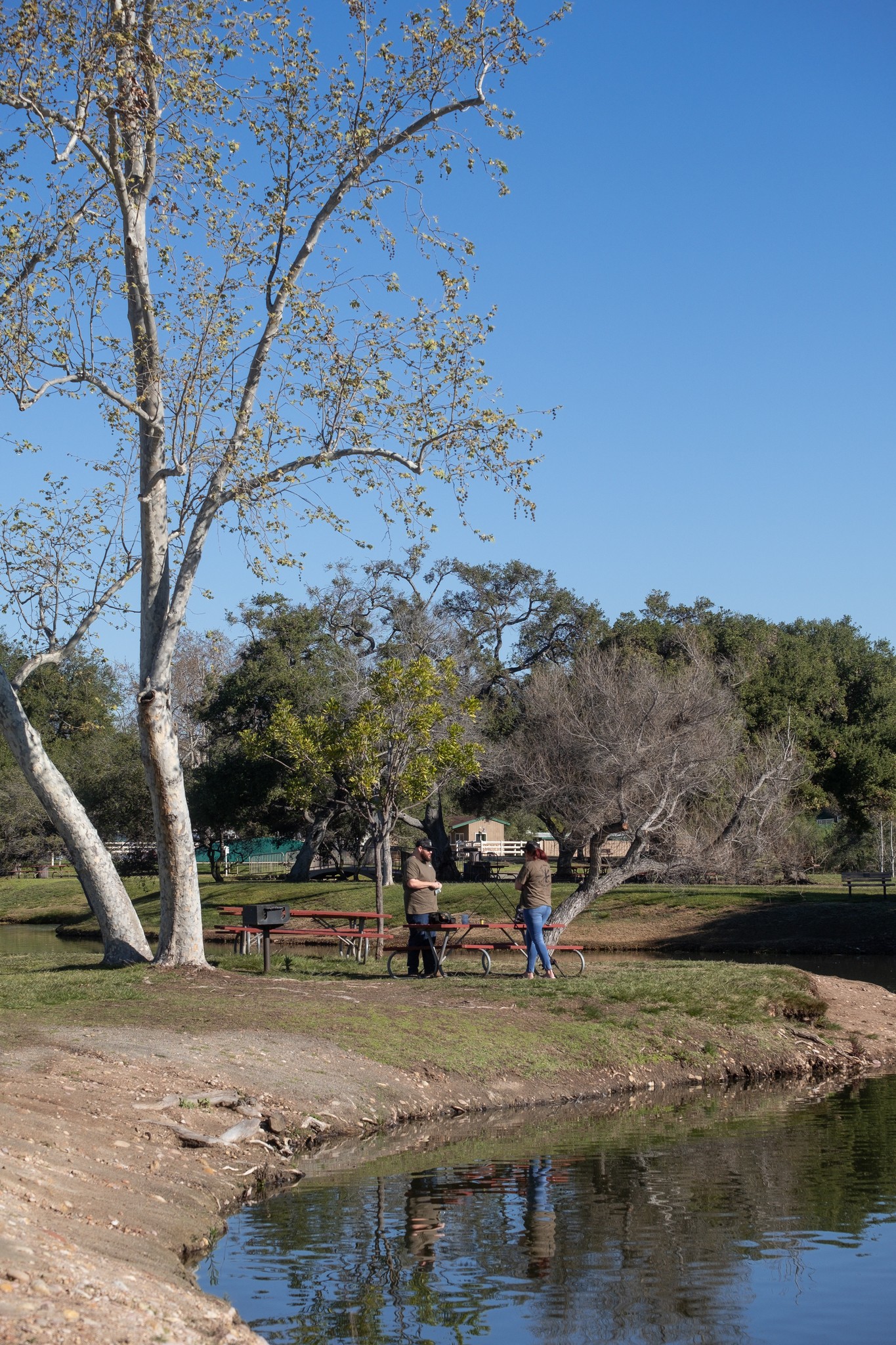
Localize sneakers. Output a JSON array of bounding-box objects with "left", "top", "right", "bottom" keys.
[
  {"left": 426, "top": 970, "right": 448, "bottom": 977},
  {"left": 408, "top": 973, "right": 422, "bottom": 978}
]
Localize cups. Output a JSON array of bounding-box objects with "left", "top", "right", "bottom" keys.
[
  {"left": 461, "top": 914, "right": 469, "bottom": 924},
  {"left": 480, "top": 919, "right": 484, "bottom": 924}
]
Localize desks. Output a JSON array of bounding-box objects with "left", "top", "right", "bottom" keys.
[{"left": 382, "top": 923, "right": 584, "bottom": 980}]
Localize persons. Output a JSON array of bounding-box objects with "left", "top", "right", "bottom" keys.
[
  {"left": 401, "top": 838, "right": 448, "bottom": 978},
  {"left": 402, "top": 1167, "right": 448, "bottom": 1273},
  {"left": 514, "top": 840, "right": 557, "bottom": 980},
  {"left": 520, "top": 1147, "right": 559, "bottom": 1283}
]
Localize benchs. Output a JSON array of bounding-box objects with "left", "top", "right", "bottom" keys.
[
  {"left": 458, "top": 864, "right": 646, "bottom": 883},
  {"left": 841, "top": 871, "right": 896, "bottom": 899},
  {"left": 382, "top": 942, "right": 518, "bottom": 979},
  {"left": 213, "top": 905, "right": 394, "bottom": 964},
  {"left": 601, "top": 848, "right": 613, "bottom": 858},
  {"left": 449, "top": 944, "right": 585, "bottom": 979}
]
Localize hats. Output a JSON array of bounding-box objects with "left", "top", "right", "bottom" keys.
[
  {"left": 519, "top": 840, "right": 540, "bottom": 850},
  {"left": 416, "top": 838, "right": 437, "bottom": 849}
]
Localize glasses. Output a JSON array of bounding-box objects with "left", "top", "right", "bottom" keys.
[{"left": 422, "top": 847, "right": 432, "bottom": 852}]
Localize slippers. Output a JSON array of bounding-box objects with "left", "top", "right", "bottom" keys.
[
  {"left": 517, "top": 975, "right": 534, "bottom": 979},
  {"left": 539, "top": 974, "right": 556, "bottom": 979}
]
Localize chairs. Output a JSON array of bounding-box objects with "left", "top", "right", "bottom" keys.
[{"left": 8, "top": 864, "right": 73, "bottom": 879}]
